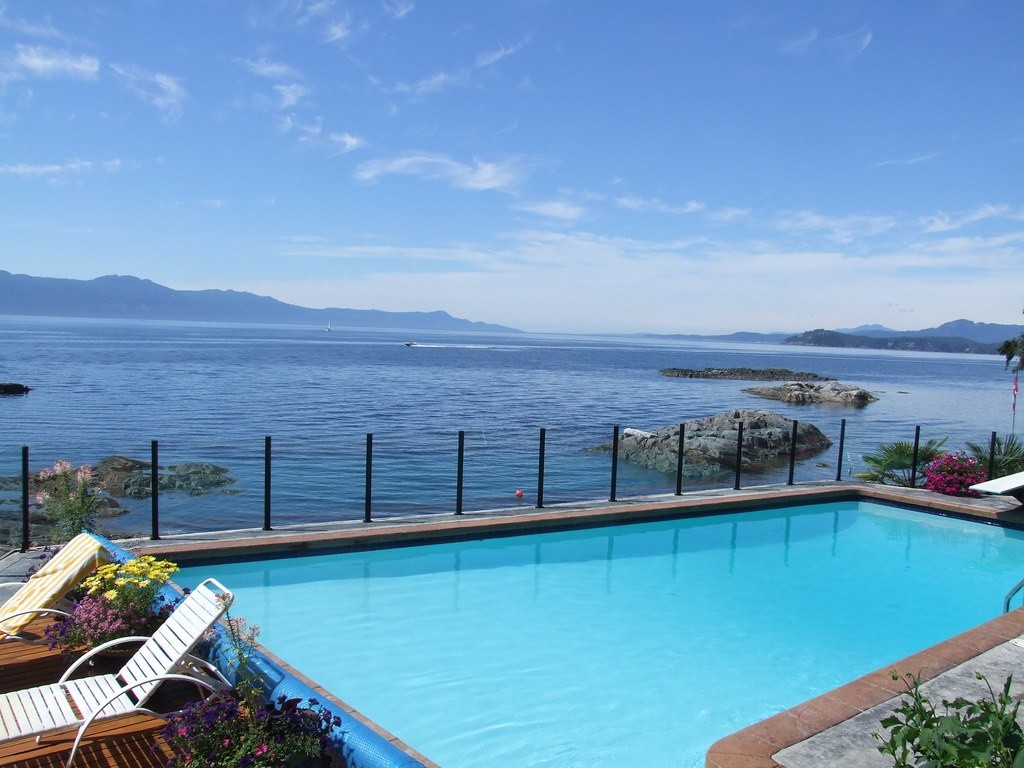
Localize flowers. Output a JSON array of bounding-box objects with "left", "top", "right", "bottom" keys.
[
  {"left": 43, "top": 555, "right": 191, "bottom": 670},
  {"left": 924, "top": 448, "right": 986, "bottom": 497},
  {"left": 149, "top": 591, "right": 345, "bottom": 768}
]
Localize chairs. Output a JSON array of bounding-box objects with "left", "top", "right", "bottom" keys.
[
  {"left": 0, "top": 577, "right": 234, "bottom": 768},
  {"left": 0, "top": 533, "right": 110, "bottom": 645}
]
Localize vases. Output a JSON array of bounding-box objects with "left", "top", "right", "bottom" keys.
[{"left": 87, "top": 646, "right": 143, "bottom": 675}]
